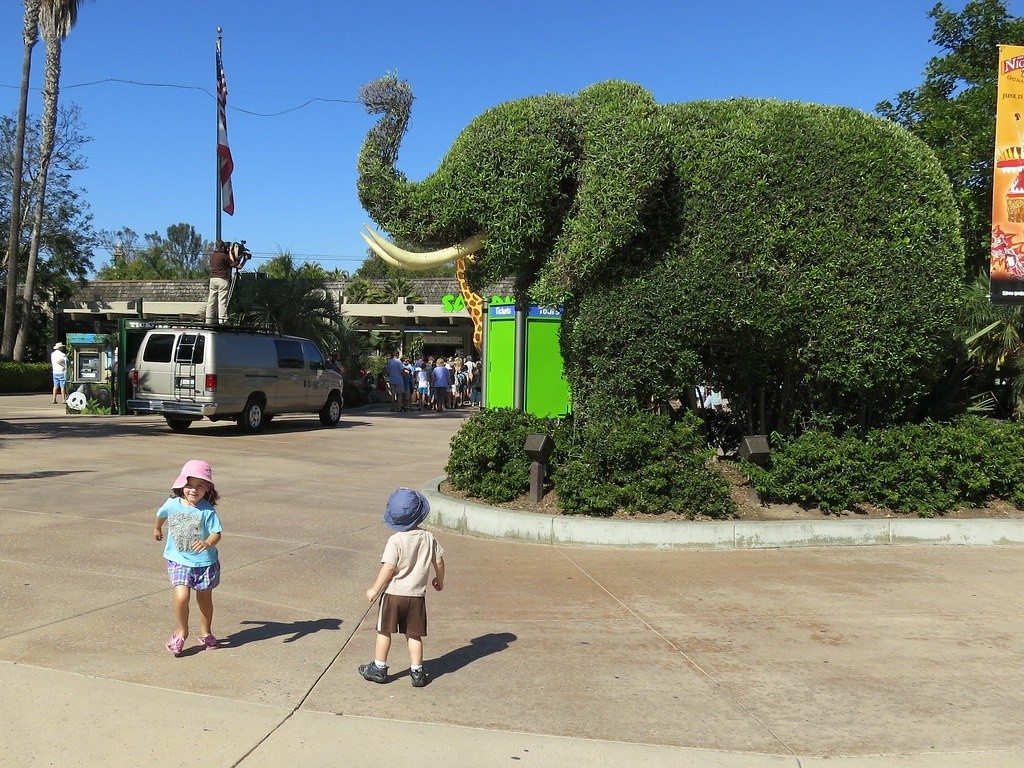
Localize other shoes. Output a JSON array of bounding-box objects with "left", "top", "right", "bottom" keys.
[{"left": 52, "top": 400, "right": 66, "bottom": 404}]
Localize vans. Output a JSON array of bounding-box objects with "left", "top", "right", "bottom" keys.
[{"left": 127, "top": 321, "right": 345, "bottom": 432}]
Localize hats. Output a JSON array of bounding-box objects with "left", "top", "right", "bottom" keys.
[
  {"left": 384, "top": 488, "right": 430, "bottom": 533},
  {"left": 172, "top": 460, "right": 214, "bottom": 497},
  {"left": 53, "top": 342, "right": 65, "bottom": 349}
]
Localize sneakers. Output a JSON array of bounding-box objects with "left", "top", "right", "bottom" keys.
[
  {"left": 409, "top": 668, "right": 427, "bottom": 687},
  {"left": 359, "top": 662, "right": 388, "bottom": 683}
]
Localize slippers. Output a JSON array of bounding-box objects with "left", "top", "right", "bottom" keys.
[
  {"left": 198, "top": 635, "right": 220, "bottom": 651},
  {"left": 165, "top": 631, "right": 189, "bottom": 654}
]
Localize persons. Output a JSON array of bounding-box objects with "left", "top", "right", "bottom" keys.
[
  {"left": 357, "top": 488, "right": 446, "bottom": 688},
  {"left": 386, "top": 350, "right": 482, "bottom": 412},
  {"left": 50, "top": 342, "right": 66, "bottom": 404},
  {"left": 154, "top": 460, "right": 222, "bottom": 655}
]
